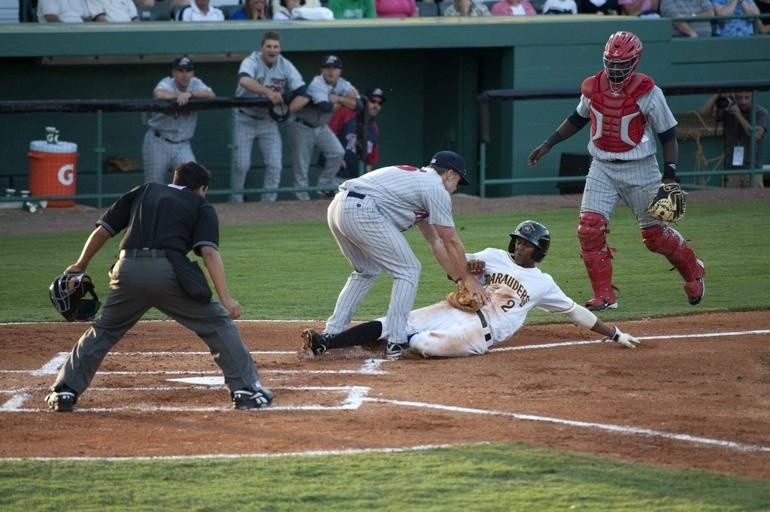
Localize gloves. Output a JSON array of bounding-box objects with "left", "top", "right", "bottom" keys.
[{"left": 613, "top": 324, "right": 640, "bottom": 350}]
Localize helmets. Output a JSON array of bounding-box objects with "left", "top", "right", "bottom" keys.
[
  {"left": 603, "top": 31, "right": 643, "bottom": 92},
  {"left": 49, "top": 271, "right": 102, "bottom": 320},
  {"left": 508, "top": 220, "right": 551, "bottom": 262},
  {"left": 646, "top": 184, "right": 689, "bottom": 222}
]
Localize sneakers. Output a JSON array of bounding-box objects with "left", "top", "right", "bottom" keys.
[
  {"left": 386, "top": 341, "right": 411, "bottom": 360},
  {"left": 44, "top": 383, "right": 76, "bottom": 412},
  {"left": 232, "top": 381, "right": 274, "bottom": 408},
  {"left": 301, "top": 328, "right": 330, "bottom": 355},
  {"left": 688, "top": 259, "right": 706, "bottom": 305},
  {"left": 587, "top": 301, "right": 617, "bottom": 311}
]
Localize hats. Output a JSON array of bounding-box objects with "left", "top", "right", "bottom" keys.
[
  {"left": 268, "top": 102, "right": 291, "bottom": 124},
  {"left": 366, "top": 88, "right": 387, "bottom": 100},
  {"left": 430, "top": 151, "right": 470, "bottom": 189},
  {"left": 321, "top": 54, "right": 341, "bottom": 67},
  {"left": 174, "top": 56, "right": 193, "bottom": 70}
]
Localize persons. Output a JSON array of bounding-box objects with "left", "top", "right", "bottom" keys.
[
  {"left": 322, "top": 151, "right": 491, "bottom": 360},
  {"left": 700, "top": 90, "right": 770, "bottom": 188},
  {"left": 295, "top": 220, "right": 641, "bottom": 359},
  {"left": 228, "top": 30, "right": 310, "bottom": 202},
  {"left": 315, "top": 86, "right": 388, "bottom": 197},
  {"left": 43, "top": 161, "right": 274, "bottom": 413},
  {"left": 527, "top": 31, "right": 706, "bottom": 311},
  {"left": 36, "top": 0, "right": 770, "bottom": 40},
  {"left": 140, "top": 55, "right": 216, "bottom": 184},
  {"left": 284, "top": 53, "right": 364, "bottom": 201}
]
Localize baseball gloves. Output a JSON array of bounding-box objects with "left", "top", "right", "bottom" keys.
[
  {"left": 448, "top": 284, "right": 484, "bottom": 311},
  {"left": 647, "top": 179, "right": 688, "bottom": 222}
]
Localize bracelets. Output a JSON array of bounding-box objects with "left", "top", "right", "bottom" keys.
[
  {"left": 187, "top": 88, "right": 194, "bottom": 98},
  {"left": 661, "top": 159, "right": 677, "bottom": 179},
  {"left": 542, "top": 129, "right": 562, "bottom": 150},
  {"left": 454, "top": 277, "right": 462, "bottom": 283}
]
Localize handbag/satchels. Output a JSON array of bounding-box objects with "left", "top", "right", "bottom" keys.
[{"left": 169, "top": 251, "right": 213, "bottom": 303}]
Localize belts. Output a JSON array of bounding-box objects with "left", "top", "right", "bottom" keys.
[
  {"left": 154, "top": 132, "right": 189, "bottom": 145},
  {"left": 476, "top": 308, "right": 494, "bottom": 349},
  {"left": 119, "top": 249, "right": 166, "bottom": 259},
  {"left": 611, "top": 157, "right": 634, "bottom": 163},
  {"left": 296, "top": 117, "right": 327, "bottom": 128},
  {"left": 238, "top": 108, "right": 268, "bottom": 121},
  {"left": 346, "top": 191, "right": 365, "bottom": 200}
]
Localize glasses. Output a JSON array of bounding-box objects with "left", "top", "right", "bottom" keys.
[
  {"left": 173, "top": 102, "right": 179, "bottom": 120},
  {"left": 371, "top": 99, "right": 384, "bottom": 105}
]
